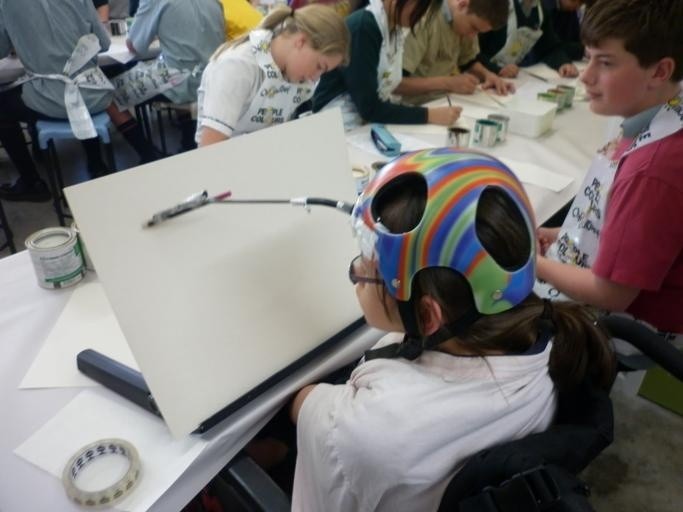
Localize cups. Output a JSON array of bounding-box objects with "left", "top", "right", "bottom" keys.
[
  {"left": 444, "top": 126, "right": 469, "bottom": 146},
  {"left": 108, "top": 17, "right": 133, "bottom": 35},
  {"left": 485, "top": 113, "right": 509, "bottom": 141},
  {"left": 536, "top": 83, "right": 576, "bottom": 113},
  {"left": 471, "top": 119, "right": 498, "bottom": 147}
]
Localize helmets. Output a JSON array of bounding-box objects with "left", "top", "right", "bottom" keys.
[{"left": 349, "top": 147, "right": 538, "bottom": 317}]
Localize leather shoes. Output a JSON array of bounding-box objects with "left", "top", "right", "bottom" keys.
[{"left": 1, "top": 178, "right": 52, "bottom": 204}]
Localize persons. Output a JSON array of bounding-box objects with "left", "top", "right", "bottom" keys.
[
  {"left": 532, "top": 1, "right": 683, "bottom": 371},
  {"left": 309, "top": 0, "right": 463, "bottom": 127},
  {"left": 393, "top": 0, "right": 516, "bottom": 98},
  {"left": 477, "top": 0, "right": 586, "bottom": 76},
  {"left": 290, "top": 147, "right": 618, "bottom": 511},
  {"left": 0, "top": 0, "right": 165, "bottom": 202},
  {"left": 105, "top": 0, "right": 227, "bottom": 161},
  {"left": 194, "top": 3, "right": 352, "bottom": 148}
]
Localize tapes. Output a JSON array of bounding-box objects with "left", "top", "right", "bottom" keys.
[{"left": 63, "top": 439, "right": 141, "bottom": 506}]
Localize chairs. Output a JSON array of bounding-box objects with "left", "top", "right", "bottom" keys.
[{"left": 586, "top": 313, "right": 682, "bottom": 512}]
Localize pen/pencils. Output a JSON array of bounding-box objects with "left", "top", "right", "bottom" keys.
[
  {"left": 445, "top": 94, "right": 452, "bottom": 105},
  {"left": 526, "top": 71, "right": 547, "bottom": 82},
  {"left": 150, "top": 189, "right": 232, "bottom": 228}
]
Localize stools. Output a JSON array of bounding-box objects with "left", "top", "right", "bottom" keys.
[
  {"left": 0, "top": 206, "right": 17, "bottom": 256},
  {"left": 151, "top": 101, "right": 198, "bottom": 158},
  {"left": 36, "top": 110, "right": 116, "bottom": 227}
]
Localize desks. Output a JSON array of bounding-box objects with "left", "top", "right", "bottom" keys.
[
  {"left": 0, "top": 61, "right": 624, "bottom": 511},
  {"left": 1, "top": 31, "right": 160, "bottom": 84}
]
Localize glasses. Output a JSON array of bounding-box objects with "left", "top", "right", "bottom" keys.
[{"left": 348, "top": 255, "right": 384, "bottom": 284}]
